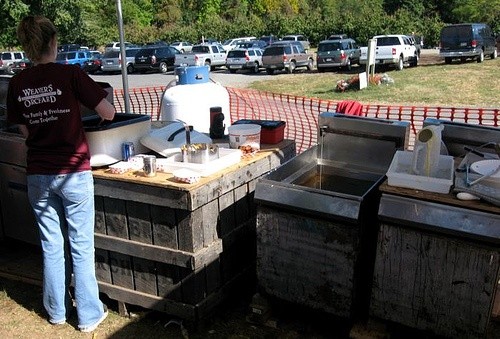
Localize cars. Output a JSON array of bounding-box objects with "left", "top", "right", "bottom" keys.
[
  {"left": 170, "top": 41, "right": 194, "bottom": 54},
  {"left": 225, "top": 48, "right": 265, "bottom": 74},
  {"left": 12, "top": 56, "right": 32, "bottom": 73}
]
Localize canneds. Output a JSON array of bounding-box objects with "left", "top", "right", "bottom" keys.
[
  {"left": 122, "top": 142, "right": 134, "bottom": 161},
  {"left": 143, "top": 155, "right": 156, "bottom": 176}
]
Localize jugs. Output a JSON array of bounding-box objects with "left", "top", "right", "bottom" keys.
[{"left": 410, "top": 124, "right": 445, "bottom": 177}]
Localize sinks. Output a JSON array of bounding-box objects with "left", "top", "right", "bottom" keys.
[{"left": 253, "top": 142, "right": 388, "bottom": 223}]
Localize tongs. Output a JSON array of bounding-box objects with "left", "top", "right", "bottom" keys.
[{"left": 466, "top": 160, "right": 500, "bottom": 188}]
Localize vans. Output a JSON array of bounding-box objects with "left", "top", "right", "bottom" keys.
[
  {"left": 281, "top": 34, "right": 311, "bottom": 50},
  {"left": 438, "top": 22, "right": 498, "bottom": 63},
  {"left": 222, "top": 36, "right": 259, "bottom": 51},
  {"left": 328, "top": 34, "right": 348, "bottom": 40},
  {"left": 100, "top": 47, "right": 143, "bottom": 72}
]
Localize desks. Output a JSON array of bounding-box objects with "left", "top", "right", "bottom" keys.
[{"left": 0, "top": 140, "right": 295, "bottom": 332}]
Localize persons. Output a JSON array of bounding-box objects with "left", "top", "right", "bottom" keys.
[{"left": 7, "top": 16, "right": 116, "bottom": 333}]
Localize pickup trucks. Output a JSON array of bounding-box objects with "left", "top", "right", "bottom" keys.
[
  {"left": 174, "top": 44, "right": 229, "bottom": 72},
  {"left": 359, "top": 33, "right": 419, "bottom": 71},
  {"left": 57, "top": 49, "right": 97, "bottom": 71},
  {"left": 408, "top": 36, "right": 422, "bottom": 58}
]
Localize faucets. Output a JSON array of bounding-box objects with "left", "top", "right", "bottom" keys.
[{"left": 319, "top": 124, "right": 329, "bottom": 137}]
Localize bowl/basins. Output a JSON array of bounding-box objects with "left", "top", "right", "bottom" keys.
[
  {"left": 228, "top": 124, "right": 262, "bottom": 153},
  {"left": 181, "top": 142, "right": 219, "bottom": 164},
  {"left": 470, "top": 159, "right": 500, "bottom": 178},
  {"left": 108, "top": 157, "right": 143, "bottom": 173},
  {"left": 173, "top": 169, "right": 200, "bottom": 184}
]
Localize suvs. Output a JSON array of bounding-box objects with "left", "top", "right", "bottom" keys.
[
  {"left": 314, "top": 38, "right": 362, "bottom": 71},
  {"left": 0, "top": 51, "right": 27, "bottom": 74},
  {"left": 105, "top": 42, "right": 133, "bottom": 52},
  {"left": 260, "top": 35, "right": 280, "bottom": 45},
  {"left": 262, "top": 44, "right": 315, "bottom": 74},
  {"left": 135, "top": 46, "right": 183, "bottom": 73}
]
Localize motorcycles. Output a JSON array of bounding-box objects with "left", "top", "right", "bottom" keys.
[{"left": 83, "top": 60, "right": 103, "bottom": 74}]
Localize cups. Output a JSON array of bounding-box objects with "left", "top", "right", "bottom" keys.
[
  {"left": 210, "top": 107, "right": 224, "bottom": 139},
  {"left": 143, "top": 155, "right": 157, "bottom": 177}
]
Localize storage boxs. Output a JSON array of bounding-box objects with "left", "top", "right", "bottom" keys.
[
  {"left": 161, "top": 149, "right": 240, "bottom": 177},
  {"left": 387, "top": 151, "right": 454, "bottom": 193},
  {"left": 233, "top": 119, "right": 286, "bottom": 145}
]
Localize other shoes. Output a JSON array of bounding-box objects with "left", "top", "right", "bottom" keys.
[{"left": 79, "top": 304, "right": 109, "bottom": 333}]
